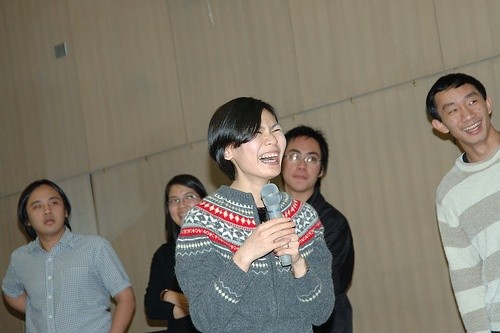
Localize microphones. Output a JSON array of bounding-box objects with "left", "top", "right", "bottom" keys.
[{"left": 260, "top": 183, "right": 297, "bottom": 267}]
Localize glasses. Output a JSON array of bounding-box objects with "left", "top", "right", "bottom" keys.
[
  {"left": 284, "top": 153, "right": 318, "bottom": 163},
  {"left": 168, "top": 194, "right": 196, "bottom": 206}
]
